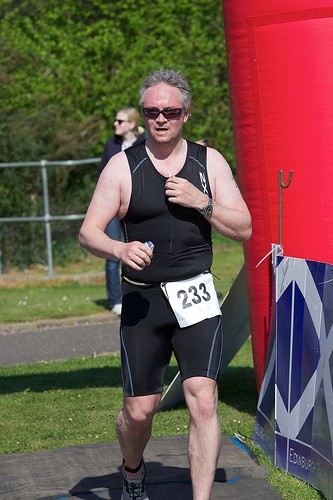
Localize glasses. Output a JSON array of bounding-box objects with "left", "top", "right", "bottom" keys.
[
  {"left": 114, "top": 119, "right": 130, "bottom": 125},
  {"left": 143, "top": 107, "right": 186, "bottom": 119}
]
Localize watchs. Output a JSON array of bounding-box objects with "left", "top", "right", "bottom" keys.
[{"left": 200, "top": 198, "right": 213, "bottom": 216}]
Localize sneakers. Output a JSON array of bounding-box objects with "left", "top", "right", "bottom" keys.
[
  {"left": 120, "top": 460, "right": 150, "bottom": 500},
  {"left": 111, "top": 304, "right": 122, "bottom": 315}
]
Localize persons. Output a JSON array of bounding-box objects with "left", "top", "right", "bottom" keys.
[
  {"left": 96, "top": 108, "right": 207, "bottom": 315},
  {"left": 80, "top": 71, "right": 253, "bottom": 500}
]
